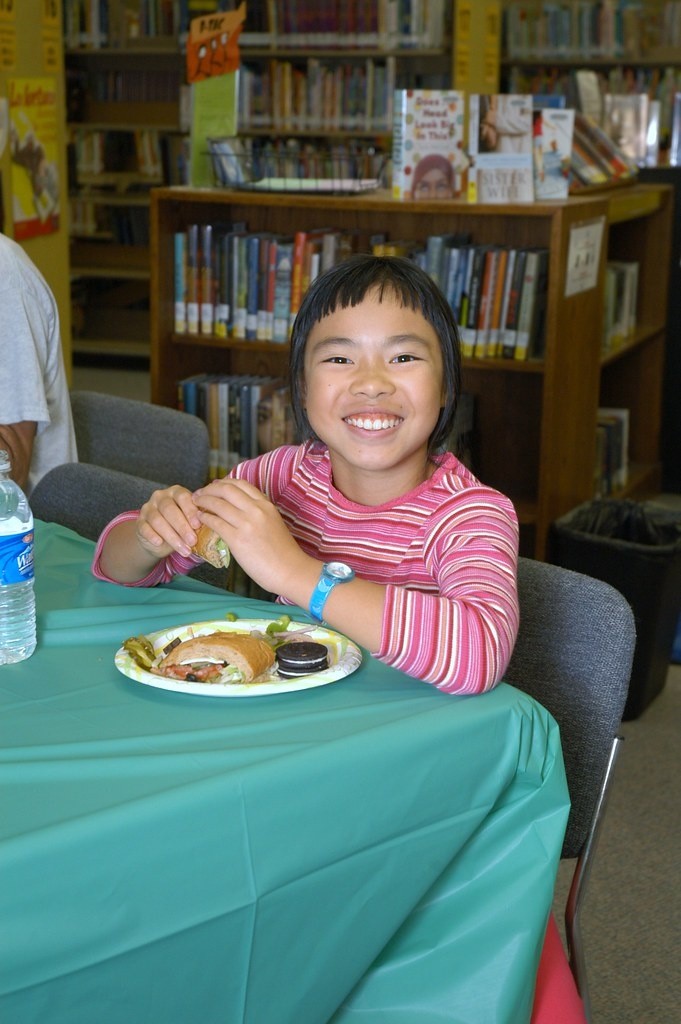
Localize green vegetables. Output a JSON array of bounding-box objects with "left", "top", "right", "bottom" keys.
[
  {"left": 265, "top": 615, "right": 293, "bottom": 654},
  {"left": 122, "top": 633, "right": 156, "bottom": 671}
]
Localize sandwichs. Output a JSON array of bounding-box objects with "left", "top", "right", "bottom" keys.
[
  {"left": 191, "top": 506, "right": 231, "bottom": 569},
  {"left": 155, "top": 631, "right": 276, "bottom": 686}
]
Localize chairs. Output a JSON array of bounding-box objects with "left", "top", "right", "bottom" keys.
[
  {"left": 485, "top": 551, "right": 636, "bottom": 1024},
  {"left": 23, "top": 385, "right": 229, "bottom": 591}
]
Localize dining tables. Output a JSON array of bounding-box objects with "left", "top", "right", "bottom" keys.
[{"left": 0, "top": 515, "right": 572, "bottom": 1022}]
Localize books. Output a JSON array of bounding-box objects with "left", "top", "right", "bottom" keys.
[
  {"left": 73, "top": 128, "right": 189, "bottom": 188},
  {"left": 597, "top": 408, "right": 629, "bottom": 498},
  {"left": 391, "top": 88, "right": 575, "bottom": 204},
  {"left": 65, "top": 68, "right": 181, "bottom": 101},
  {"left": 178, "top": 57, "right": 397, "bottom": 131},
  {"left": 605, "top": 261, "right": 639, "bottom": 349},
  {"left": 173, "top": 221, "right": 549, "bottom": 361},
  {"left": 176, "top": 372, "right": 298, "bottom": 481},
  {"left": 501, "top": 0, "right": 681, "bottom": 60},
  {"left": 71, "top": 202, "right": 114, "bottom": 240},
  {"left": 241, "top": 136, "right": 390, "bottom": 188},
  {"left": 500, "top": 65, "right": 681, "bottom": 151},
  {"left": 63, "top": 0, "right": 445, "bottom": 52}
]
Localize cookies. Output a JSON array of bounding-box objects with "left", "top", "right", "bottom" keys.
[{"left": 275, "top": 642, "right": 328, "bottom": 679}]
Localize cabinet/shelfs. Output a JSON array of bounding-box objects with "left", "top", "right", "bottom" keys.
[{"left": 0, "top": 0, "right": 681, "bottom": 567}]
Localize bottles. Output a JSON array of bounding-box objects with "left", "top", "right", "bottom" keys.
[{"left": 0, "top": 449, "right": 37, "bottom": 665}]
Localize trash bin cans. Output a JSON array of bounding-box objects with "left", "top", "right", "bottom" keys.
[{"left": 548, "top": 498, "right": 681, "bottom": 723}]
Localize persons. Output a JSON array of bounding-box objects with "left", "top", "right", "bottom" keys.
[
  {"left": 0, "top": 233, "right": 80, "bottom": 503},
  {"left": 91, "top": 252, "right": 519, "bottom": 694},
  {"left": 532, "top": 110, "right": 556, "bottom": 182}
]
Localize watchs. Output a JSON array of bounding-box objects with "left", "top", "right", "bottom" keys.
[{"left": 303, "top": 561, "right": 355, "bottom": 625}]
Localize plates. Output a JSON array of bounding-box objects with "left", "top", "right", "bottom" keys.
[{"left": 114, "top": 617, "right": 363, "bottom": 696}]
